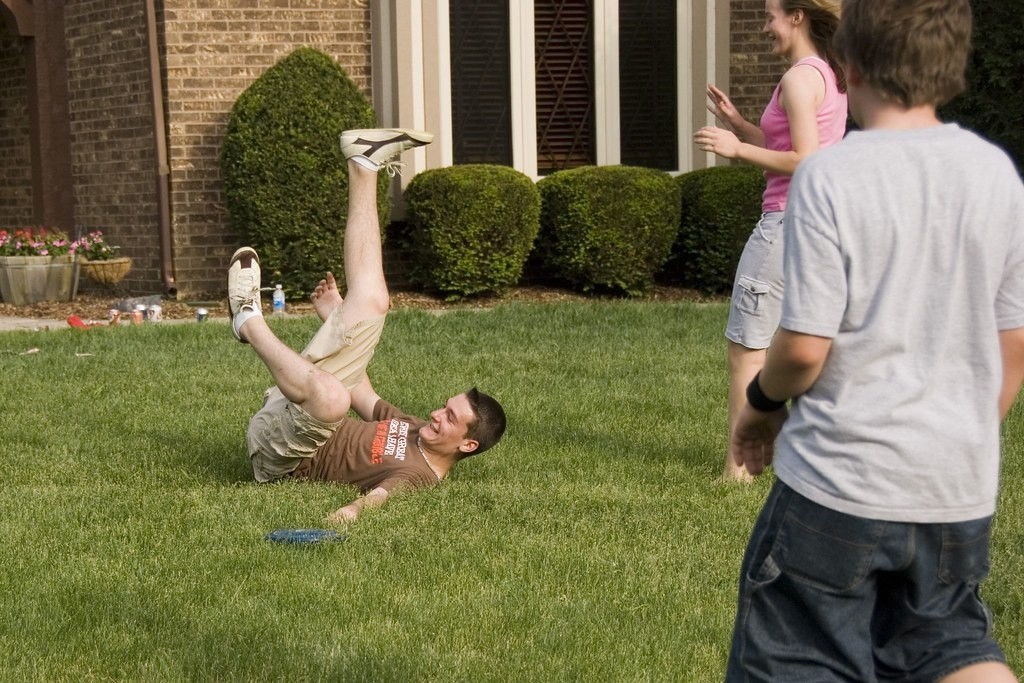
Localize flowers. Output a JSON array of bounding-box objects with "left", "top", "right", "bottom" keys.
[
  {"left": 0, "top": 225, "right": 78, "bottom": 256},
  {"left": 74, "top": 231, "right": 122, "bottom": 260}
]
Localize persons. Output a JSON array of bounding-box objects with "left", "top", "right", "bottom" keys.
[
  {"left": 694, "top": 0, "right": 848, "bottom": 488},
  {"left": 228, "top": 130, "right": 506, "bottom": 528},
  {"left": 722, "top": 0, "right": 1024, "bottom": 683}
]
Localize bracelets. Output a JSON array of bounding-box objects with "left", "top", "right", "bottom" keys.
[{"left": 746, "top": 369, "right": 787, "bottom": 413}]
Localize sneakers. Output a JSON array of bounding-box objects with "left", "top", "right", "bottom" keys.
[
  {"left": 340, "top": 128, "right": 433, "bottom": 178},
  {"left": 226, "top": 246, "right": 262, "bottom": 344}
]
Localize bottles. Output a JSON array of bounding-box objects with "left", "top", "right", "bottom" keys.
[{"left": 273, "top": 284, "right": 285, "bottom": 313}]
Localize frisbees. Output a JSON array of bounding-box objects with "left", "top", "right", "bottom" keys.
[{"left": 266, "top": 529, "right": 347, "bottom": 543}]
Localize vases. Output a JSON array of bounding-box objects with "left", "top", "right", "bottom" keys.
[
  {"left": 81, "top": 256, "right": 132, "bottom": 285},
  {"left": 0, "top": 254, "right": 82, "bottom": 306}
]
